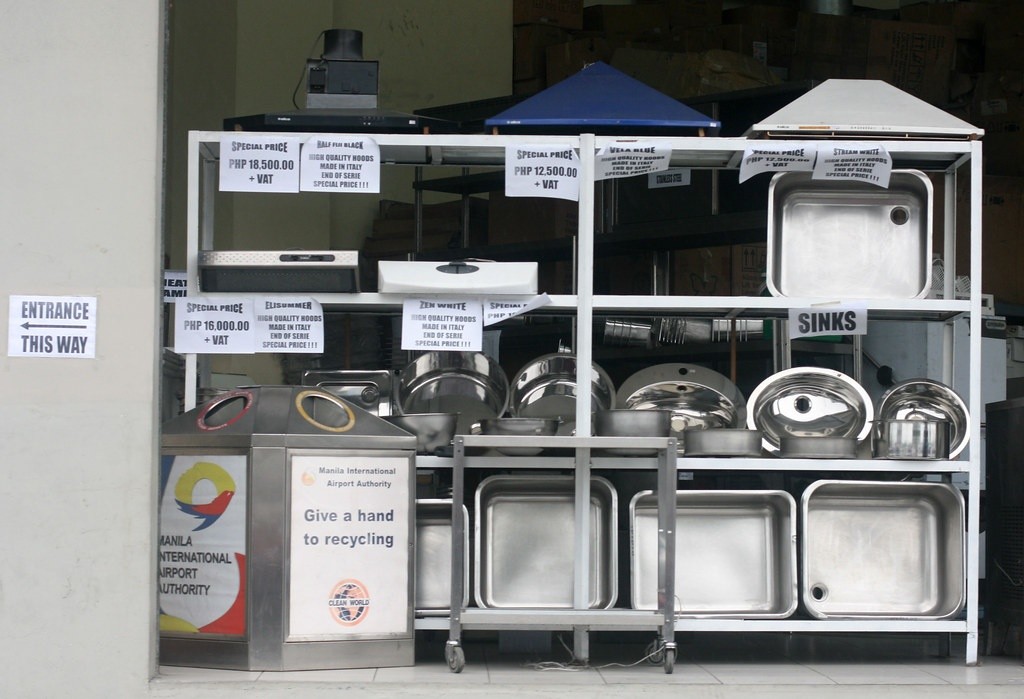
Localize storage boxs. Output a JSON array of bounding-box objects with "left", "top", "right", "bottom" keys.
[
  {"left": 1007, "top": 326, "right": 1024, "bottom": 379},
  {"left": 363, "top": 1, "right": 1024, "bottom": 306}
]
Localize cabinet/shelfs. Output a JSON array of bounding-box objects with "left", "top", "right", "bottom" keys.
[
  {"left": 184, "top": 131, "right": 982, "bottom": 666},
  {"left": 406, "top": 79, "right": 855, "bottom": 359}
]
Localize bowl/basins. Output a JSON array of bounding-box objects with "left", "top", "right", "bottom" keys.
[{"left": 302, "top": 357, "right": 972, "bottom": 457}]
